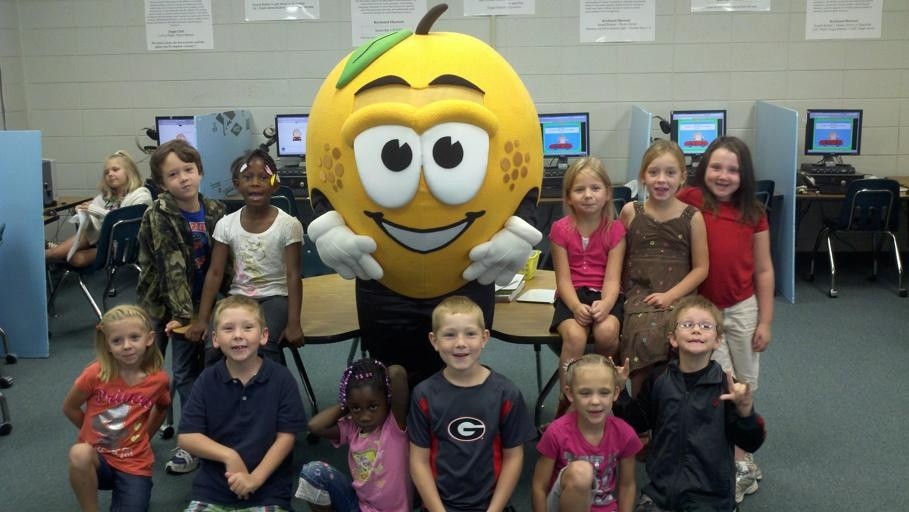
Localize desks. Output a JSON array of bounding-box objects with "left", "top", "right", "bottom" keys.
[
  {"left": 219, "top": 190, "right": 312, "bottom": 203},
  {"left": 159, "top": 267, "right": 622, "bottom": 445},
  {"left": 796, "top": 177, "right": 909, "bottom": 247},
  {"left": 537, "top": 183, "right": 626, "bottom": 205}
]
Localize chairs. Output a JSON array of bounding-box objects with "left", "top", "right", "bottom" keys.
[
  {"left": 610, "top": 187, "right": 631, "bottom": 221},
  {"left": 44, "top": 178, "right": 159, "bottom": 320},
  {"left": 0, "top": 223, "right": 17, "bottom": 438},
  {"left": 807, "top": 179, "right": 908, "bottom": 298},
  {"left": 270, "top": 185, "right": 298, "bottom": 219},
  {"left": 755, "top": 179, "right": 775, "bottom": 223}
]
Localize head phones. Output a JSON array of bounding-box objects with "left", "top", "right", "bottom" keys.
[
  {"left": 263, "top": 121, "right": 277, "bottom": 152},
  {"left": 134, "top": 124, "right": 159, "bottom": 154},
  {"left": 647, "top": 112, "right": 670, "bottom": 144}
]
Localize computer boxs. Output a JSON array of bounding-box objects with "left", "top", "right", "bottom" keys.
[
  {"left": 543, "top": 167, "right": 575, "bottom": 189},
  {"left": 42, "top": 158, "right": 58, "bottom": 203},
  {"left": 277, "top": 166, "right": 307, "bottom": 188},
  {"left": 797, "top": 169, "right": 864, "bottom": 190}
]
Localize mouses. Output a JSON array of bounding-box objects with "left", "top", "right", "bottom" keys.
[{"left": 44, "top": 210, "right": 57, "bottom": 216}]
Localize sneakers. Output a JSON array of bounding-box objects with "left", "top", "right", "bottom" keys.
[
  {"left": 735, "top": 452, "right": 762, "bottom": 503},
  {"left": 165, "top": 448, "right": 199, "bottom": 475}
]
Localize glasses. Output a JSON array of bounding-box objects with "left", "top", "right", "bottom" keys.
[{"left": 673, "top": 320, "right": 716, "bottom": 332}]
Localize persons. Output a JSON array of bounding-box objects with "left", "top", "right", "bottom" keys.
[
  {"left": 184, "top": 150, "right": 304, "bottom": 370},
  {"left": 289, "top": 356, "right": 418, "bottom": 512},
  {"left": 404, "top": 294, "right": 539, "bottom": 512},
  {"left": 60, "top": 302, "right": 170, "bottom": 512},
  {"left": 618, "top": 138, "right": 710, "bottom": 400},
  {"left": 674, "top": 134, "right": 776, "bottom": 505},
  {"left": 45, "top": 150, "right": 153, "bottom": 270},
  {"left": 174, "top": 292, "right": 308, "bottom": 512},
  {"left": 548, "top": 154, "right": 630, "bottom": 408},
  {"left": 529, "top": 353, "right": 644, "bottom": 512},
  {"left": 135, "top": 139, "right": 229, "bottom": 475},
  {"left": 607, "top": 293, "right": 768, "bottom": 511}
]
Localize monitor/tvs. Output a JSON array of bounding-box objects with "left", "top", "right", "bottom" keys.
[
  {"left": 805, "top": 108, "right": 863, "bottom": 167},
  {"left": 538, "top": 112, "right": 590, "bottom": 168},
  {"left": 670, "top": 109, "right": 727, "bottom": 157},
  {"left": 154, "top": 114, "right": 196, "bottom": 154},
  {"left": 274, "top": 112, "right": 311, "bottom": 159}
]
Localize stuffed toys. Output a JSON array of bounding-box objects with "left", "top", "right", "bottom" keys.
[{"left": 303, "top": 4, "right": 546, "bottom": 389}]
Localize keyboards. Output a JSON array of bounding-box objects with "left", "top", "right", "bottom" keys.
[
  {"left": 804, "top": 162, "right": 858, "bottom": 176},
  {"left": 683, "top": 161, "right": 705, "bottom": 185},
  {"left": 276, "top": 185, "right": 309, "bottom": 198}
]
